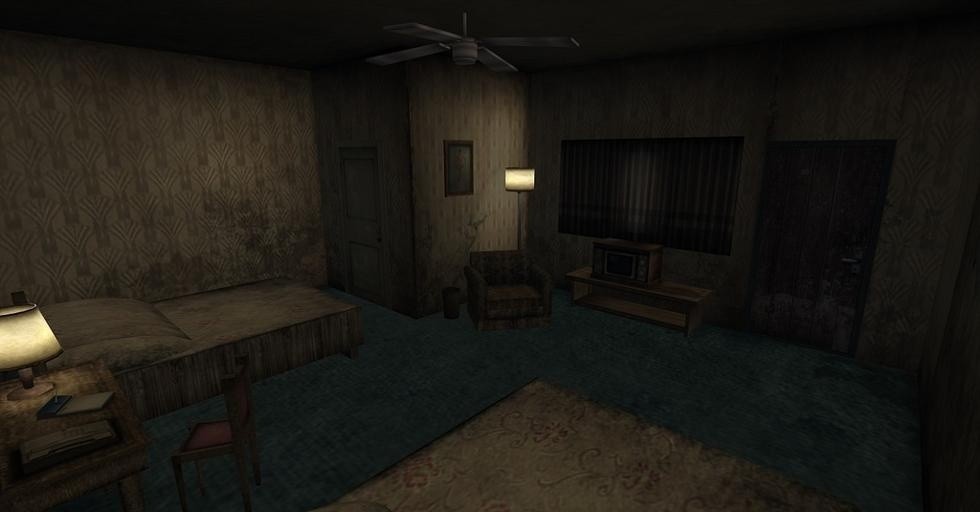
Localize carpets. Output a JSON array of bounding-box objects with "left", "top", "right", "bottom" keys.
[{"left": 300, "top": 375, "right": 868, "bottom": 512}]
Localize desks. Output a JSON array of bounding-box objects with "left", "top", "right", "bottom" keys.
[{"left": 0, "top": 359, "right": 158, "bottom": 511}]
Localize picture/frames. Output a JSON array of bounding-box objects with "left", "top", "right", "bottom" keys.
[{"left": 443, "top": 138, "right": 475, "bottom": 196}]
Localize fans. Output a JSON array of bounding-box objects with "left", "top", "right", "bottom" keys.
[{"left": 361, "top": 10, "right": 585, "bottom": 76}]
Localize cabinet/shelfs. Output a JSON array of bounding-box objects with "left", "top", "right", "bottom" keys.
[{"left": 567, "top": 266, "right": 716, "bottom": 333}]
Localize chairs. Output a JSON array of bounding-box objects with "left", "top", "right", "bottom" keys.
[
  {"left": 169, "top": 352, "right": 267, "bottom": 512},
  {"left": 463, "top": 249, "right": 557, "bottom": 333}
]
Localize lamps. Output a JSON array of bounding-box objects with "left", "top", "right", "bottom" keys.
[
  {"left": 503, "top": 166, "right": 537, "bottom": 252},
  {"left": 1, "top": 303, "right": 65, "bottom": 400}
]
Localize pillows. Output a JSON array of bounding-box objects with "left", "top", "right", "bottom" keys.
[{"left": 34, "top": 297, "right": 192, "bottom": 372}]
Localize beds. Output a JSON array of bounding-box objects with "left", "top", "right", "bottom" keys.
[{"left": 9, "top": 275, "right": 367, "bottom": 424}]
